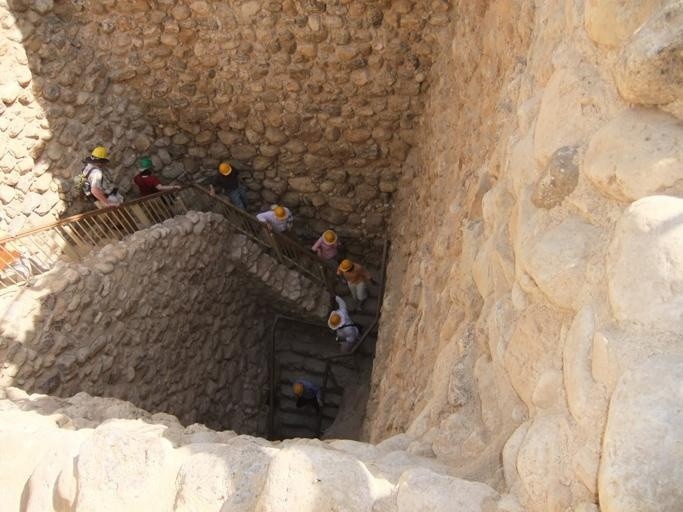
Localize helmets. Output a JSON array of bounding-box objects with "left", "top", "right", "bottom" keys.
[
  {"left": 139, "top": 160, "right": 152, "bottom": 168},
  {"left": 275, "top": 207, "right": 286, "bottom": 219},
  {"left": 322, "top": 230, "right": 338, "bottom": 246},
  {"left": 340, "top": 259, "right": 352, "bottom": 272},
  {"left": 219, "top": 164, "right": 232, "bottom": 176},
  {"left": 92, "top": 147, "right": 111, "bottom": 159},
  {"left": 328, "top": 310, "right": 345, "bottom": 330},
  {"left": 293, "top": 384, "right": 304, "bottom": 397}
]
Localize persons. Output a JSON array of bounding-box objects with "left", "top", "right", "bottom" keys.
[
  {"left": 328, "top": 295, "right": 362, "bottom": 354},
  {"left": 81, "top": 147, "right": 124, "bottom": 210},
  {"left": 209, "top": 162, "right": 249, "bottom": 211},
  {"left": 256, "top": 204, "right": 294, "bottom": 234},
  {"left": 131, "top": 157, "right": 182, "bottom": 206},
  {"left": 287, "top": 377, "right": 324, "bottom": 417},
  {"left": 336, "top": 259, "right": 372, "bottom": 305},
  {"left": 311, "top": 229, "right": 345, "bottom": 262}
]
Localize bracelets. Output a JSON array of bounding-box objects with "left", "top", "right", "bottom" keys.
[{"left": 173, "top": 185, "right": 177, "bottom": 189}]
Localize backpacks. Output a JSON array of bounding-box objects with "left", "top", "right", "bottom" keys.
[{"left": 73, "top": 168, "right": 104, "bottom": 202}]
